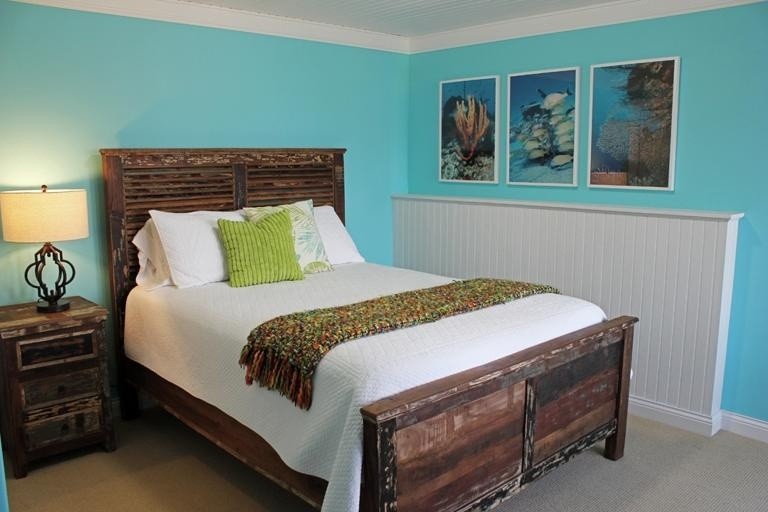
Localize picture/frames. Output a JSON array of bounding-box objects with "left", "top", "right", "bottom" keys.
[
  {"left": 585, "top": 55, "right": 681, "bottom": 192},
  {"left": 504, "top": 65, "right": 581, "bottom": 187},
  {"left": 437, "top": 74, "right": 501, "bottom": 185}
]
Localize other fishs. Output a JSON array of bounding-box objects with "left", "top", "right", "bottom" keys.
[
  {"left": 509, "top": 106, "right": 573, "bottom": 168},
  {"left": 539, "top": 90, "right": 572, "bottom": 112}
]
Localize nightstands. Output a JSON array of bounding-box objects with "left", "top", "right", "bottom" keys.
[{"left": 0, "top": 294, "right": 119, "bottom": 480}]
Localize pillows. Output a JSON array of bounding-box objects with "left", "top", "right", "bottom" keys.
[
  {"left": 146, "top": 209, "right": 249, "bottom": 290},
  {"left": 240, "top": 204, "right": 364, "bottom": 268},
  {"left": 130, "top": 218, "right": 175, "bottom": 291},
  {"left": 217, "top": 210, "right": 303, "bottom": 289},
  {"left": 241, "top": 198, "right": 334, "bottom": 274}
]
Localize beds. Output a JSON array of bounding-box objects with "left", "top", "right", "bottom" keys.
[{"left": 96, "top": 146, "right": 639, "bottom": 511}]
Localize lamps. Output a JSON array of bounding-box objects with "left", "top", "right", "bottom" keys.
[{"left": 0, "top": 184, "right": 89, "bottom": 314}]
